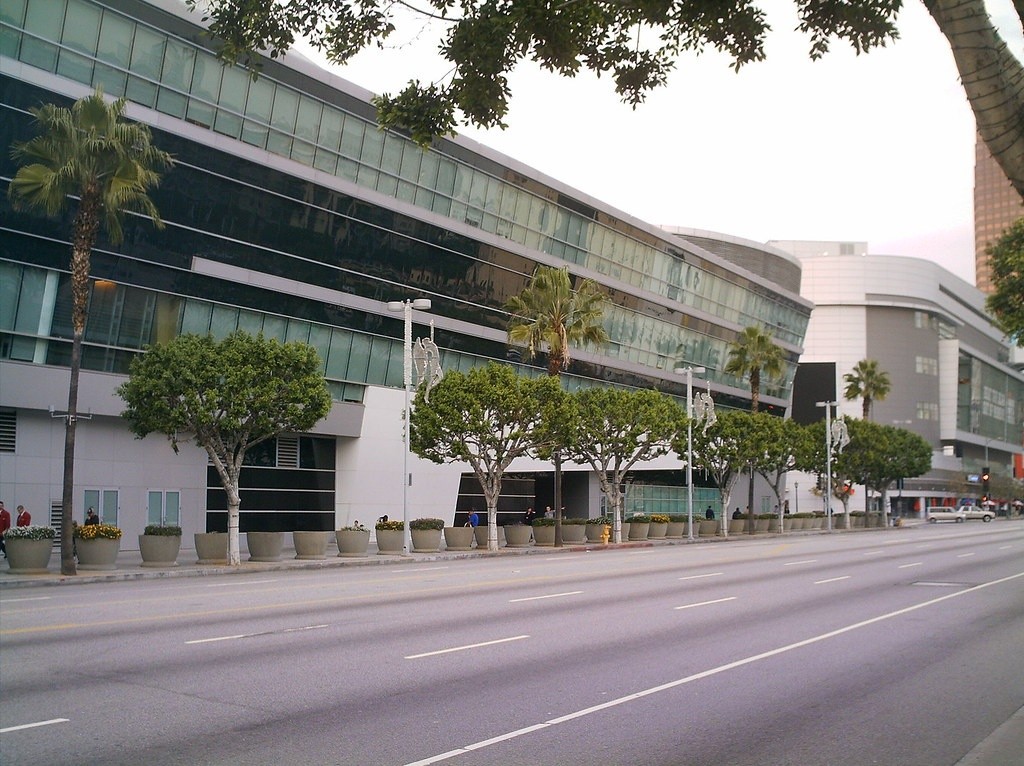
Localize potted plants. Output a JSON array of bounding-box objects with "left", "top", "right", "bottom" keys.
[
  {"left": 625, "top": 515, "right": 650, "bottom": 541},
  {"left": 137, "top": 526, "right": 183, "bottom": 567},
  {"left": 561, "top": 517, "right": 585, "bottom": 545},
  {"left": 728, "top": 509, "right": 892, "bottom": 536}
]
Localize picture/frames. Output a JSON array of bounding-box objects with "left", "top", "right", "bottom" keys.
[{"left": 410, "top": 528, "right": 441, "bottom": 553}]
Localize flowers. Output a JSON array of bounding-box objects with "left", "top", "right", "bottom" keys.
[
  {"left": 409, "top": 515, "right": 445, "bottom": 530},
  {"left": 2, "top": 525, "right": 57, "bottom": 541},
  {"left": 376, "top": 518, "right": 405, "bottom": 532},
  {"left": 532, "top": 517, "right": 559, "bottom": 527},
  {"left": 650, "top": 513, "right": 670, "bottom": 523},
  {"left": 73, "top": 523, "right": 123, "bottom": 542},
  {"left": 585, "top": 514, "right": 611, "bottom": 525}
]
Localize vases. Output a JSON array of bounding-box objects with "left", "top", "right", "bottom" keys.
[
  {"left": 665, "top": 521, "right": 685, "bottom": 539},
  {"left": 1, "top": 540, "right": 58, "bottom": 575},
  {"left": 533, "top": 525, "right": 556, "bottom": 546},
  {"left": 503, "top": 524, "right": 533, "bottom": 548},
  {"left": 444, "top": 527, "right": 475, "bottom": 551},
  {"left": 193, "top": 533, "right": 228, "bottom": 564},
  {"left": 376, "top": 529, "right": 404, "bottom": 554},
  {"left": 585, "top": 524, "right": 606, "bottom": 543},
  {"left": 293, "top": 531, "right": 330, "bottom": 560},
  {"left": 683, "top": 520, "right": 701, "bottom": 538},
  {"left": 73, "top": 536, "right": 121, "bottom": 571},
  {"left": 699, "top": 519, "right": 720, "bottom": 538},
  {"left": 647, "top": 521, "right": 668, "bottom": 540},
  {"left": 475, "top": 525, "right": 503, "bottom": 549},
  {"left": 247, "top": 531, "right": 286, "bottom": 562},
  {"left": 336, "top": 530, "right": 371, "bottom": 557},
  {"left": 609, "top": 523, "right": 630, "bottom": 542}
]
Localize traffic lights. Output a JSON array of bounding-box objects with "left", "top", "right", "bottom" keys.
[
  {"left": 982, "top": 474, "right": 989, "bottom": 492},
  {"left": 982, "top": 495, "right": 988, "bottom": 501},
  {"left": 982, "top": 466, "right": 990, "bottom": 475}
]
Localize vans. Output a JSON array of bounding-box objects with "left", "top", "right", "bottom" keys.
[{"left": 927, "top": 506, "right": 966, "bottom": 523}]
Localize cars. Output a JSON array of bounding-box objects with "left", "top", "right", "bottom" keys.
[{"left": 957, "top": 505, "right": 996, "bottom": 522}]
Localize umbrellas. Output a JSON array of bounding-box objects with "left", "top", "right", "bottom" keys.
[
  {"left": 1013, "top": 500, "right": 1024, "bottom": 506},
  {"left": 981, "top": 499, "right": 996, "bottom": 506}
]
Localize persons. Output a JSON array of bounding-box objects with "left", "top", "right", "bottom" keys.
[
  {"left": 524, "top": 507, "right": 534, "bottom": 525},
  {"left": 732, "top": 506, "right": 750, "bottom": 517},
  {"left": 774, "top": 505, "right": 779, "bottom": 512},
  {"left": 466, "top": 508, "right": 479, "bottom": 527},
  {"left": 544, "top": 505, "right": 565, "bottom": 518},
  {"left": 0, "top": 501, "right": 11, "bottom": 559},
  {"left": 16, "top": 505, "right": 31, "bottom": 527},
  {"left": 705, "top": 505, "right": 715, "bottom": 519},
  {"left": 84, "top": 507, "right": 99, "bottom": 526}
]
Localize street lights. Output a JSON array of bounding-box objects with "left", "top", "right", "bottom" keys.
[
  {"left": 387, "top": 298, "right": 432, "bottom": 558},
  {"left": 986, "top": 437, "right": 1004, "bottom": 501},
  {"left": 815, "top": 399, "right": 841, "bottom": 531},
  {"left": 673, "top": 366, "right": 708, "bottom": 540}
]
